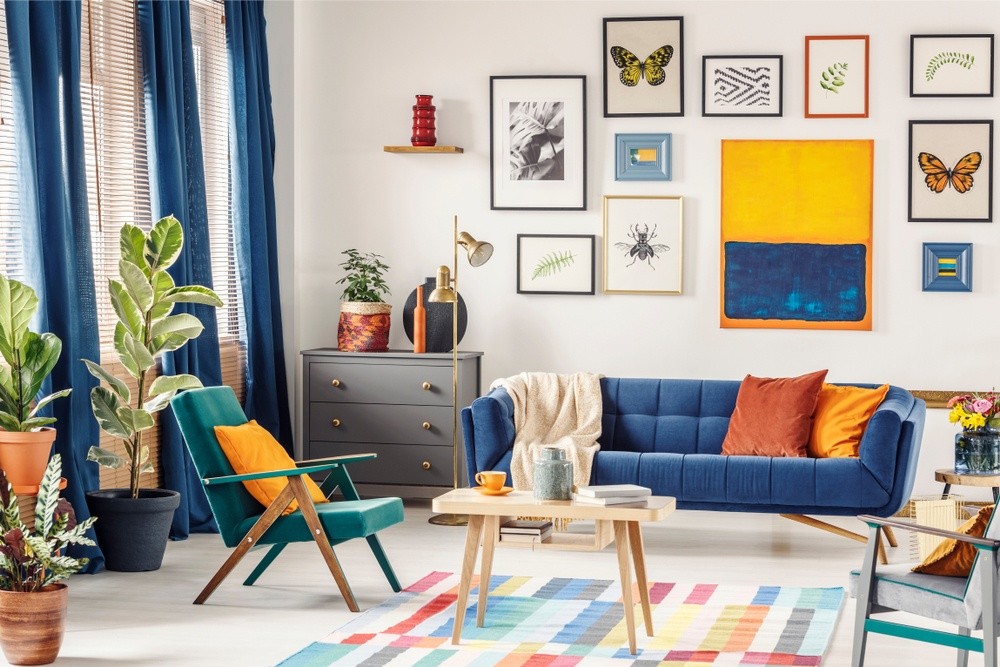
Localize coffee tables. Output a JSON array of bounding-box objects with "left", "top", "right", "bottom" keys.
[
  {"left": 430, "top": 487, "right": 678, "bottom": 658},
  {"left": 934, "top": 469, "right": 1000, "bottom": 505}
]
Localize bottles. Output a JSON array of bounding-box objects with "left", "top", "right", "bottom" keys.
[
  {"left": 411, "top": 94, "right": 436, "bottom": 146},
  {"left": 413, "top": 287, "right": 425, "bottom": 353}
]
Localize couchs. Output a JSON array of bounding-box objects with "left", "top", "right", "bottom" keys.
[{"left": 461, "top": 369, "right": 928, "bottom": 566}]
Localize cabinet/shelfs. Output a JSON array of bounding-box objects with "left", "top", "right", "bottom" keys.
[{"left": 298, "top": 348, "right": 485, "bottom": 500}]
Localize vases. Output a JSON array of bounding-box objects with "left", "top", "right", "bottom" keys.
[{"left": 954, "top": 426, "right": 1000, "bottom": 476}]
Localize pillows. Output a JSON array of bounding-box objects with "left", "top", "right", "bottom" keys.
[
  {"left": 806, "top": 383, "right": 888, "bottom": 458},
  {"left": 910, "top": 505, "right": 995, "bottom": 576},
  {"left": 211, "top": 419, "right": 329, "bottom": 516},
  {"left": 722, "top": 370, "right": 829, "bottom": 458}
]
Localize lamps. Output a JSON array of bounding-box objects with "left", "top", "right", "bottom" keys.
[{"left": 427, "top": 215, "right": 494, "bottom": 525}]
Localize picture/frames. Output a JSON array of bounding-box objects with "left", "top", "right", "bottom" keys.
[
  {"left": 614, "top": 132, "right": 672, "bottom": 182},
  {"left": 804, "top": 36, "right": 869, "bottom": 118},
  {"left": 602, "top": 16, "right": 685, "bottom": 117},
  {"left": 908, "top": 120, "right": 993, "bottom": 222},
  {"left": 922, "top": 242, "right": 972, "bottom": 292},
  {"left": 910, "top": 33, "right": 994, "bottom": 96},
  {"left": 516, "top": 233, "right": 596, "bottom": 295},
  {"left": 701, "top": 55, "right": 783, "bottom": 117},
  {"left": 602, "top": 194, "right": 684, "bottom": 297},
  {"left": 489, "top": 75, "right": 587, "bottom": 210}
]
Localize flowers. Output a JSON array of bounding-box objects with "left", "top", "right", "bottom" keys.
[{"left": 947, "top": 387, "right": 1000, "bottom": 470}]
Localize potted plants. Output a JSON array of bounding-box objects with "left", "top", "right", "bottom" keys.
[
  {"left": 337, "top": 248, "right": 390, "bottom": 352},
  {"left": 1, "top": 454, "right": 97, "bottom": 667},
  {"left": 1, "top": 274, "right": 67, "bottom": 495},
  {"left": 84, "top": 213, "right": 225, "bottom": 572}
]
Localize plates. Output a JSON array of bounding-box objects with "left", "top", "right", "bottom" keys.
[{"left": 472, "top": 486, "right": 514, "bottom": 495}]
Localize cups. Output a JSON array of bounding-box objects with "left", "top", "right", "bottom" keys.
[{"left": 474, "top": 470, "right": 507, "bottom": 490}]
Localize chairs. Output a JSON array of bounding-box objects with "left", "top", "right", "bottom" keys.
[
  {"left": 168, "top": 386, "right": 405, "bottom": 612},
  {"left": 845, "top": 493, "right": 1000, "bottom": 667}
]
